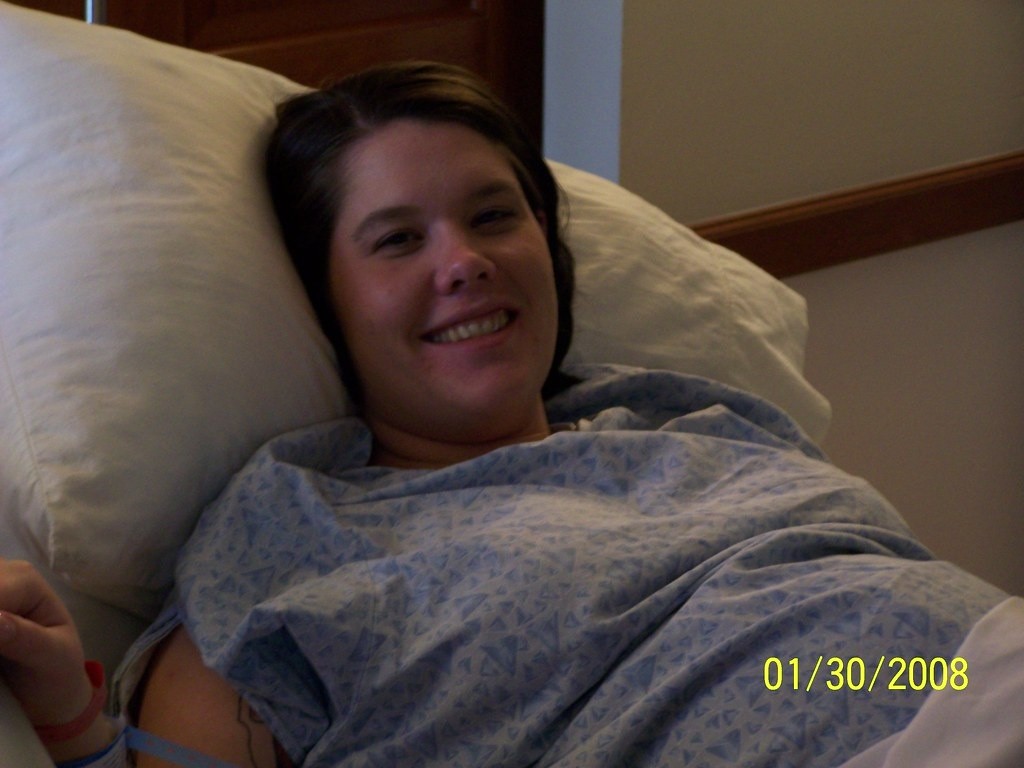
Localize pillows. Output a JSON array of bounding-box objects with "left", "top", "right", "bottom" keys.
[{"left": 0, "top": 0, "right": 834, "bottom": 623}]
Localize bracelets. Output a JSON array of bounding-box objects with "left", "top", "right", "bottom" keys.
[{"left": 32, "top": 660, "right": 130, "bottom": 768}]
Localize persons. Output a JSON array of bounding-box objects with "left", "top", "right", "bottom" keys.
[{"left": 0, "top": 61, "right": 1024, "bottom": 768}]
